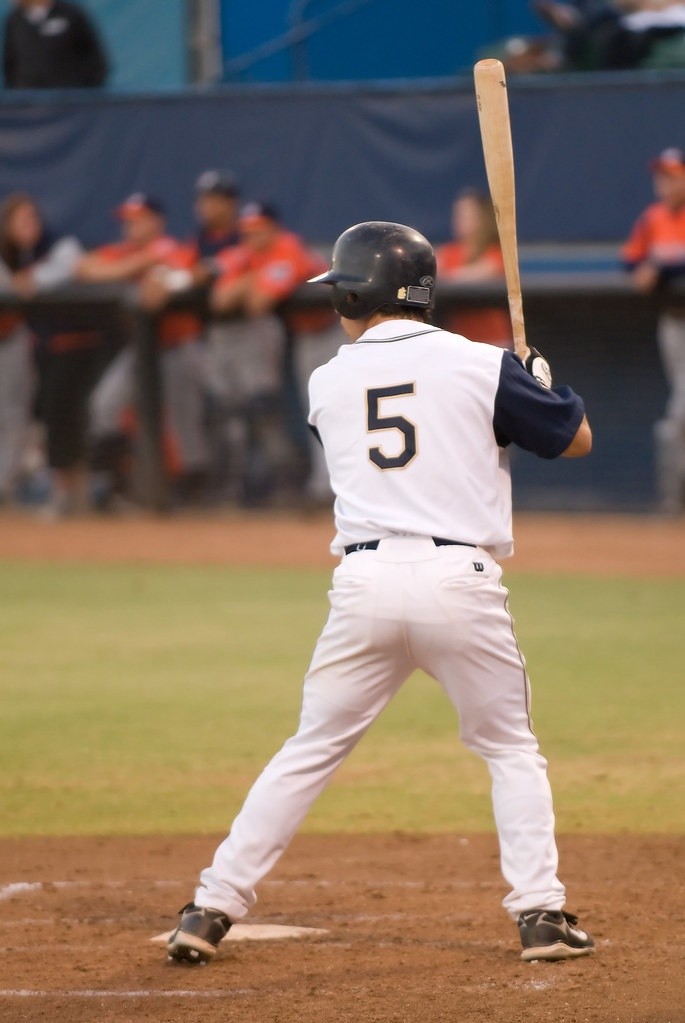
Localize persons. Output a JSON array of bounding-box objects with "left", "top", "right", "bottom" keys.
[
  {"left": 421, "top": 186, "right": 519, "bottom": 356},
  {"left": 1, "top": 171, "right": 352, "bottom": 512},
  {"left": 609, "top": 150, "right": 685, "bottom": 511},
  {"left": 172, "top": 218, "right": 595, "bottom": 974}
]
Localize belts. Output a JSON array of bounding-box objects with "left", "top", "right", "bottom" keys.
[{"left": 344, "top": 535, "right": 478, "bottom": 547}]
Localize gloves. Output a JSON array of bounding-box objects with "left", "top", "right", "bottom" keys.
[{"left": 513, "top": 345, "right": 552, "bottom": 391}]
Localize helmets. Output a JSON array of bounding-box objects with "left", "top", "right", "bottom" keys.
[
  {"left": 193, "top": 168, "right": 240, "bottom": 195},
  {"left": 305, "top": 221, "right": 438, "bottom": 320}
]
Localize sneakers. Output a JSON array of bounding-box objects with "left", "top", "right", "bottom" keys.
[
  {"left": 517, "top": 910, "right": 595, "bottom": 961},
  {"left": 167, "top": 900, "right": 234, "bottom": 963},
  {"left": 115, "top": 192, "right": 170, "bottom": 220}
]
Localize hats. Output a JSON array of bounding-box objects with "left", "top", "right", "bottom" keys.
[
  {"left": 235, "top": 201, "right": 278, "bottom": 230},
  {"left": 650, "top": 148, "right": 685, "bottom": 174}
]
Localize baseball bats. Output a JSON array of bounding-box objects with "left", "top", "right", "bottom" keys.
[{"left": 474, "top": 57, "right": 527, "bottom": 361}]
